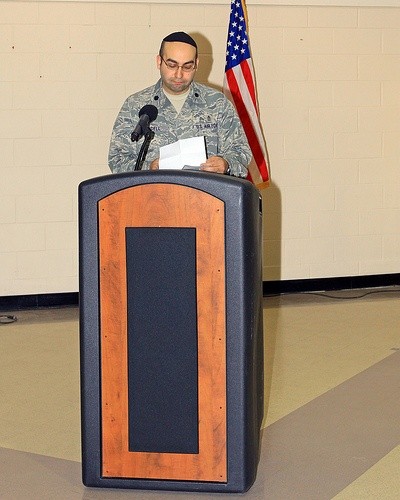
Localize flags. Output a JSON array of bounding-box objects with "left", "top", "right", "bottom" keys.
[{"left": 222, "top": 0, "right": 270, "bottom": 190}]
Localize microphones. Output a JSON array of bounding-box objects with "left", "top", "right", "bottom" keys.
[{"left": 131, "top": 104, "right": 158, "bottom": 142}]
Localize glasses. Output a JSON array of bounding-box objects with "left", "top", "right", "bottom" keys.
[{"left": 161, "top": 56, "right": 197, "bottom": 72}]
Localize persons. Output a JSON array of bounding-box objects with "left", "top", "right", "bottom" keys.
[{"left": 109, "top": 32, "right": 253, "bottom": 178}]
